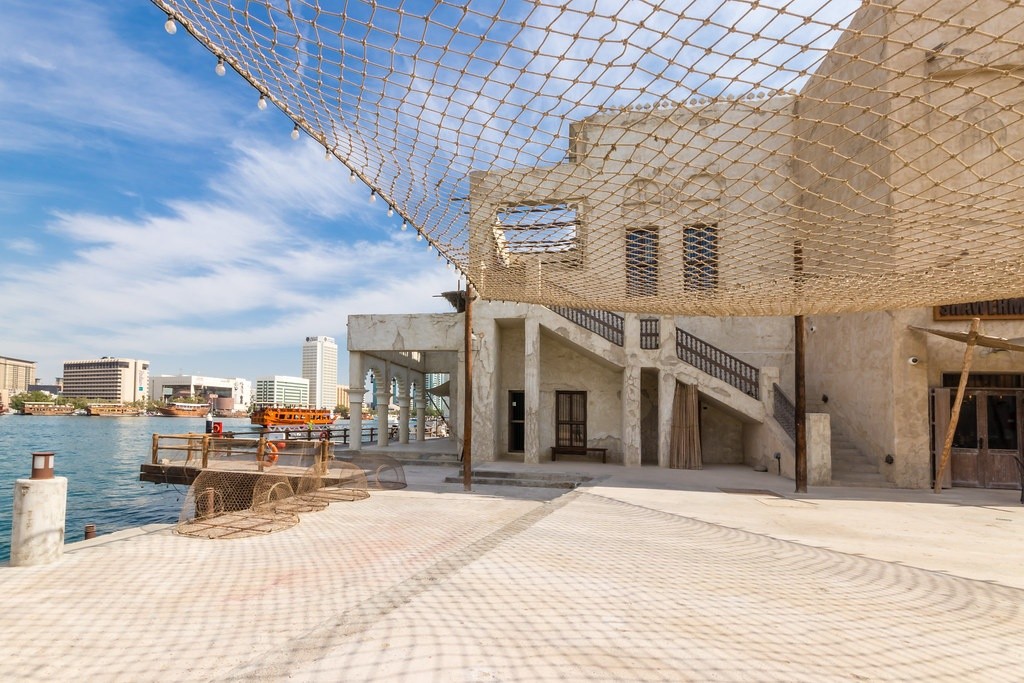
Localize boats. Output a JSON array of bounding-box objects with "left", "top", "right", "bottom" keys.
[
  {"left": 250, "top": 407, "right": 333, "bottom": 428},
  {"left": 95, "top": 407, "right": 141, "bottom": 417},
  {"left": 156, "top": 402, "right": 211, "bottom": 418},
  {"left": 344, "top": 412, "right": 374, "bottom": 419},
  {"left": 17, "top": 402, "right": 75, "bottom": 416}
]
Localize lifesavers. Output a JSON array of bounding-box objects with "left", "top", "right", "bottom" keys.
[
  {"left": 256, "top": 443, "right": 278, "bottom": 466},
  {"left": 319, "top": 431, "right": 329, "bottom": 442}
]
